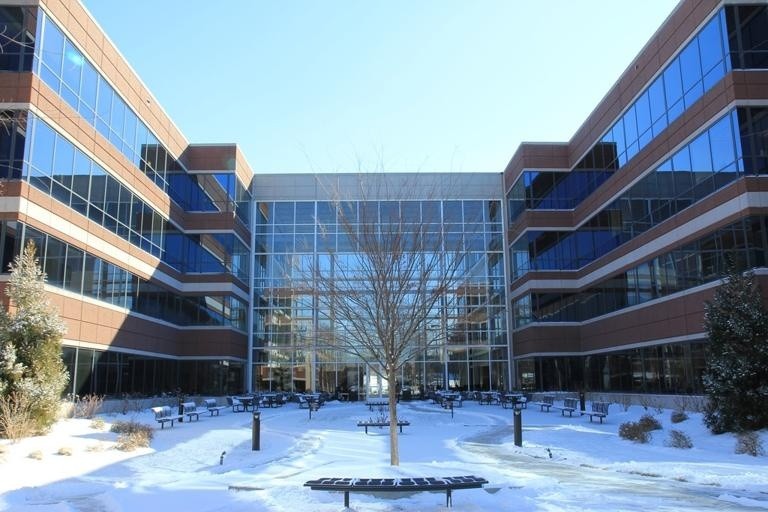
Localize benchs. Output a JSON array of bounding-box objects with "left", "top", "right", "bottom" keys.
[
  {"left": 432, "top": 389, "right": 613, "bottom": 424},
  {"left": 356, "top": 420, "right": 410, "bottom": 433},
  {"left": 365, "top": 402, "right": 390, "bottom": 411},
  {"left": 304, "top": 477, "right": 489, "bottom": 508},
  {"left": 153, "top": 392, "right": 322, "bottom": 427}
]
